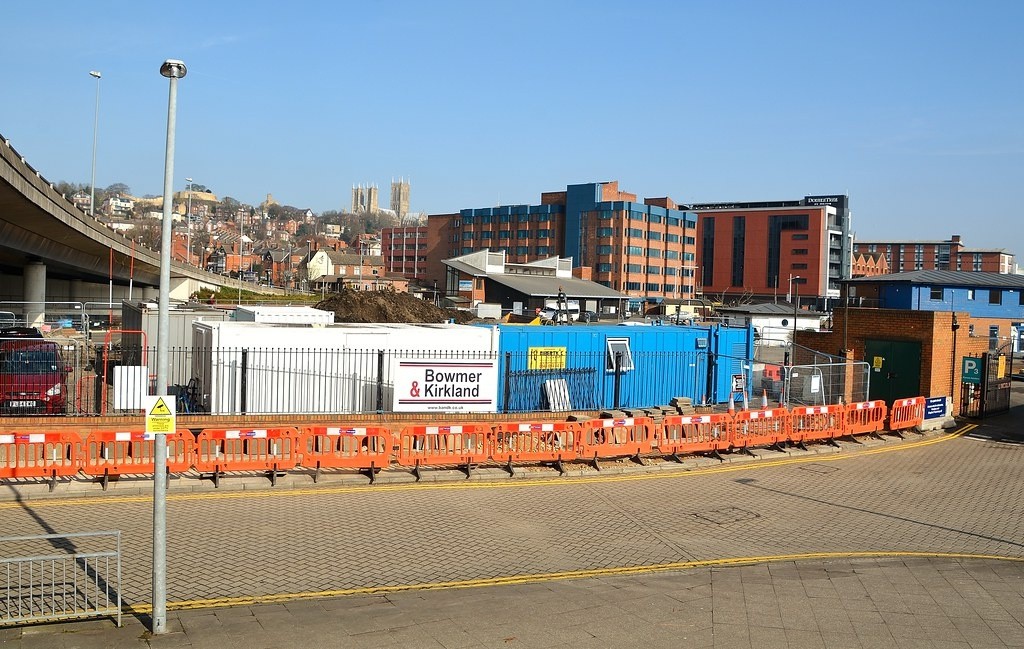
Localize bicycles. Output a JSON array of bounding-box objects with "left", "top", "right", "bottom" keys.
[{"left": 174, "top": 379, "right": 207, "bottom": 416}]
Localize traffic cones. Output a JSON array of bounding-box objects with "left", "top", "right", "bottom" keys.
[
  {"left": 702, "top": 395, "right": 706, "bottom": 407},
  {"left": 760, "top": 389, "right": 768, "bottom": 411},
  {"left": 741, "top": 391, "right": 749, "bottom": 412},
  {"left": 726, "top": 392, "right": 737, "bottom": 416},
  {"left": 778, "top": 393, "right": 783, "bottom": 408}
]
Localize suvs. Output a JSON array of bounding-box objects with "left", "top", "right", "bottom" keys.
[
  {"left": 580, "top": 311, "right": 600, "bottom": 323},
  {"left": 0, "top": 327, "right": 73, "bottom": 417},
  {"left": 666, "top": 311, "right": 692, "bottom": 323}
]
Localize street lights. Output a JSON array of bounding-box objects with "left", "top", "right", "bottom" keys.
[
  {"left": 186, "top": 178, "right": 192, "bottom": 265},
  {"left": 787, "top": 274, "right": 800, "bottom": 304},
  {"left": 238, "top": 208, "right": 245, "bottom": 280},
  {"left": 152, "top": 57, "right": 188, "bottom": 633},
  {"left": 674, "top": 265, "right": 699, "bottom": 299},
  {"left": 273, "top": 240, "right": 311, "bottom": 288},
  {"left": 360, "top": 242, "right": 363, "bottom": 291},
  {"left": 89, "top": 71, "right": 101, "bottom": 219}
]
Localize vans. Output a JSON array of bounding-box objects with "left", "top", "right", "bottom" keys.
[{"left": 537, "top": 303, "right": 580, "bottom": 323}]
